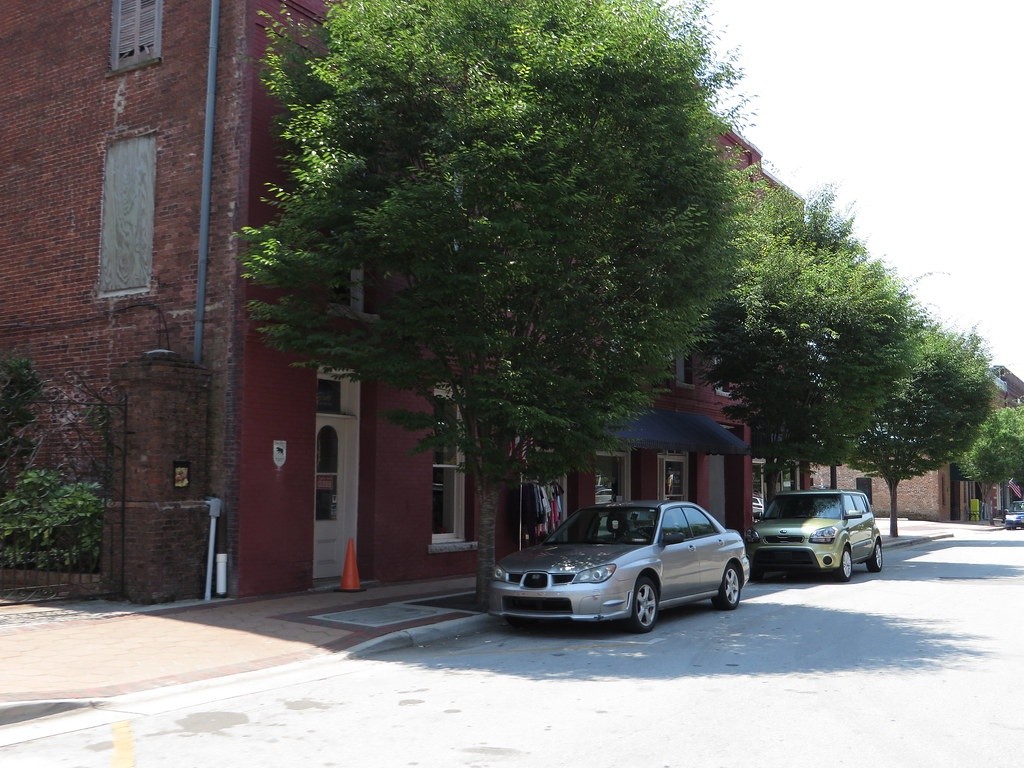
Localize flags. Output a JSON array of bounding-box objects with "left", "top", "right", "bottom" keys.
[{"left": 1007, "top": 478, "right": 1022, "bottom": 499}]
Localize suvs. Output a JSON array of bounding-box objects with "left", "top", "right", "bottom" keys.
[{"left": 747, "top": 492, "right": 883, "bottom": 580}]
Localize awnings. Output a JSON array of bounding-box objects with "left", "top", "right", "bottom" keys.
[{"left": 579, "top": 400, "right": 753, "bottom": 458}]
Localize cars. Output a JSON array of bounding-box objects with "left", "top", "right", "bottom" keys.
[
  {"left": 491, "top": 500, "right": 750, "bottom": 630},
  {"left": 1004, "top": 502, "right": 1024, "bottom": 531}
]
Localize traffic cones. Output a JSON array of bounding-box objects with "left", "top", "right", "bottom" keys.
[{"left": 341, "top": 539, "right": 360, "bottom": 592}]
